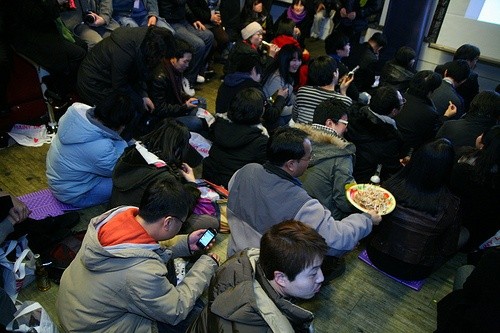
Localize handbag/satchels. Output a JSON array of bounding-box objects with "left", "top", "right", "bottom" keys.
[
  {"left": 13, "top": 249, "right": 37, "bottom": 291},
  {"left": 6, "top": 301, "right": 59, "bottom": 333},
  {"left": 7, "top": 123, "right": 55, "bottom": 147}
]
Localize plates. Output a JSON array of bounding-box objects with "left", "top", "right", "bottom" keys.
[{"left": 346, "top": 184, "right": 397, "bottom": 215}]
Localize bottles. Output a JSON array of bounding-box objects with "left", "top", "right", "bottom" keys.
[
  {"left": 261, "top": 40, "right": 281, "bottom": 53},
  {"left": 33, "top": 253, "right": 51, "bottom": 292},
  {"left": 81, "top": 14, "right": 96, "bottom": 25},
  {"left": 368, "top": 164, "right": 382, "bottom": 186},
  {"left": 337, "top": 65, "right": 360, "bottom": 85}
]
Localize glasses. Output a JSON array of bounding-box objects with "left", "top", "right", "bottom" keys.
[
  {"left": 399, "top": 98, "right": 406, "bottom": 106},
  {"left": 331, "top": 118, "right": 348, "bottom": 128},
  {"left": 294, "top": 151, "right": 315, "bottom": 161}
]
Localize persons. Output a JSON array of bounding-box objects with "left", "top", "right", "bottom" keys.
[
  {"left": 381, "top": 45, "right": 500, "bottom": 117},
  {"left": 291, "top": 98, "right": 360, "bottom": 221},
  {"left": 346, "top": 85, "right": 418, "bottom": 183},
  {"left": 226, "top": 0, "right": 388, "bottom": 124},
  {"left": 396, "top": 70, "right": 458, "bottom": 148},
  {"left": 110, "top": 118, "right": 223, "bottom": 234},
  {"left": 137, "top": 40, "right": 207, "bottom": 133},
  {"left": 365, "top": 138, "right": 474, "bottom": 281},
  {"left": 78, "top": 25, "right": 176, "bottom": 136},
  {"left": 226, "top": 128, "right": 383, "bottom": 280},
  {"left": 215, "top": 41, "right": 291, "bottom": 125},
  {"left": 46, "top": 89, "right": 153, "bottom": 207},
  {"left": 38, "top": 0, "right": 270, "bottom": 95},
  {"left": 200, "top": 85, "right": 292, "bottom": 190},
  {"left": 435, "top": 85, "right": 500, "bottom": 255},
  {"left": 206, "top": 219, "right": 328, "bottom": 333},
  {"left": 0, "top": 188, "right": 80, "bottom": 269},
  {"left": 55, "top": 171, "right": 221, "bottom": 333},
  {"left": 436, "top": 203, "right": 500, "bottom": 333}
]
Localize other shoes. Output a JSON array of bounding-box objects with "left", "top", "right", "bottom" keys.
[
  {"left": 196, "top": 74, "right": 205, "bottom": 83},
  {"left": 182, "top": 76, "right": 195, "bottom": 96}
]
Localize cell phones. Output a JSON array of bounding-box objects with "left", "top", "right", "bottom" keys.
[
  {"left": 197, "top": 227, "right": 217, "bottom": 248},
  {"left": 190, "top": 100, "right": 200, "bottom": 104}
]
